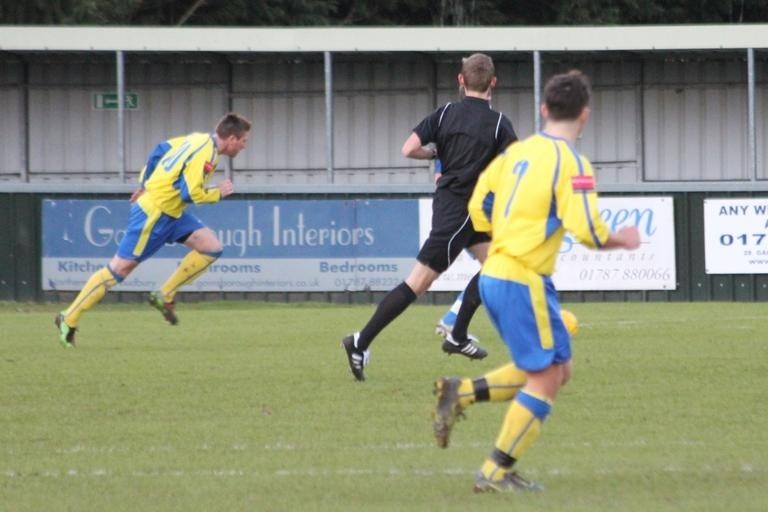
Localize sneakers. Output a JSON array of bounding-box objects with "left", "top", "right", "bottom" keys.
[
  {"left": 342, "top": 335, "right": 366, "bottom": 382},
  {"left": 433, "top": 377, "right": 465, "bottom": 449},
  {"left": 148, "top": 292, "right": 178, "bottom": 324},
  {"left": 436, "top": 320, "right": 486, "bottom": 360},
  {"left": 472, "top": 470, "right": 541, "bottom": 493},
  {"left": 54, "top": 314, "right": 75, "bottom": 348}
]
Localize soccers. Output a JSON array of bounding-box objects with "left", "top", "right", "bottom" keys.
[{"left": 560, "top": 310, "right": 578, "bottom": 335}]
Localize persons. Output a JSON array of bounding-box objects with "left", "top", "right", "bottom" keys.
[
  {"left": 53, "top": 110, "right": 253, "bottom": 349},
  {"left": 433, "top": 158, "right": 480, "bottom": 342},
  {"left": 432, "top": 70, "right": 640, "bottom": 494},
  {"left": 342, "top": 52, "right": 520, "bottom": 381}
]
